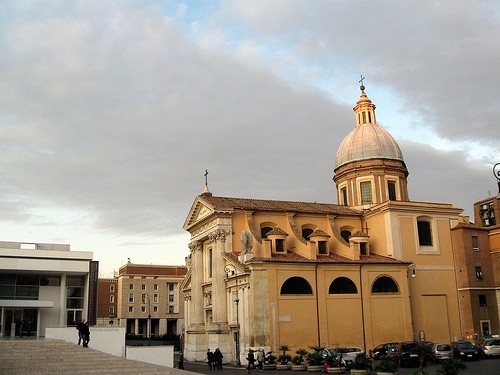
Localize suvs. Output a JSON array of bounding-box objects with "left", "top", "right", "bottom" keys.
[{"left": 368, "top": 341, "right": 423, "bottom": 367}]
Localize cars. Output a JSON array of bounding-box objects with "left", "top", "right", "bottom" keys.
[
  {"left": 450, "top": 341, "right": 480, "bottom": 362},
  {"left": 337, "top": 347, "right": 364, "bottom": 362},
  {"left": 318, "top": 348, "right": 342, "bottom": 361},
  {"left": 476, "top": 338, "right": 500, "bottom": 359},
  {"left": 432, "top": 343, "right": 456, "bottom": 360}
]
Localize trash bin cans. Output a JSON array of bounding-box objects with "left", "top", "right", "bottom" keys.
[{"left": 345, "top": 360, "right": 353, "bottom": 371}]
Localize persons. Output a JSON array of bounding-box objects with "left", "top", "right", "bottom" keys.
[
  {"left": 207, "top": 348, "right": 223, "bottom": 371},
  {"left": 179, "top": 352, "right": 184, "bottom": 370},
  {"left": 85, "top": 322, "right": 89, "bottom": 347},
  {"left": 76, "top": 319, "right": 86, "bottom": 347},
  {"left": 246, "top": 349, "right": 265, "bottom": 372},
  {"left": 27, "top": 320, "right": 32, "bottom": 338}
]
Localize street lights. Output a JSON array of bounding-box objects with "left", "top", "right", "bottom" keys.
[{"left": 223, "top": 264, "right": 242, "bottom": 368}]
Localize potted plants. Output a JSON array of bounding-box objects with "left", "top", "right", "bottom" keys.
[
  {"left": 376, "top": 354, "right": 401, "bottom": 375},
  {"left": 420, "top": 340, "right": 434, "bottom": 360},
  {"left": 306, "top": 346, "right": 326, "bottom": 372},
  {"left": 276, "top": 345, "right": 293, "bottom": 370},
  {"left": 350, "top": 353, "right": 375, "bottom": 375},
  {"left": 263, "top": 351, "right": 277, "bottom": 369},
  {"left": 323, "top": 346, "right": 347, "bottom": 373},
  {"left": 291, "top": 348, "right": 308, "bottom": 371}
]
